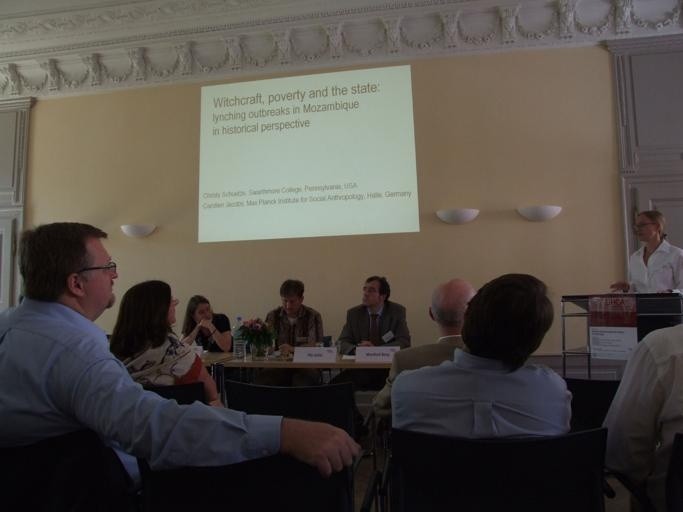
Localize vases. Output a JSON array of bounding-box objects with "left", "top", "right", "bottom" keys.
[{"left": 250, "top": 344, "right": 268, "bottom": 361}]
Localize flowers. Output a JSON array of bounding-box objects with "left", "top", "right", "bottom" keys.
[{"left": 238, "top": 316, "right": 277, "bottom": 356}]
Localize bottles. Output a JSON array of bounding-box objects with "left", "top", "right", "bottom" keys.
[{"left": 232, "top": 316, "right": 246, "bottom": 360}]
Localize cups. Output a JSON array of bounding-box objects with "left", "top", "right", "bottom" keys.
[
  {"left": 295, "top": 336, "right": 309, "bottom": 345},
  {"left": 322, "top": 335, "right": 332, "bottom": 347},
  {"left": 192, "top": 345, "right": 202, "bottom": 357}
]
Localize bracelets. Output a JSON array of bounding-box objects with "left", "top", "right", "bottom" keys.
[{"left": 210, "top": 326, "right": 217, "bottom": 337}]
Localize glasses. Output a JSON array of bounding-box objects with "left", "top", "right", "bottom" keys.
[
  {"left": 632, "top": 222, "right": 657, "bottom": 229},
  {"left": 77, "top": 261, "right": 117, "bottom": 274}
]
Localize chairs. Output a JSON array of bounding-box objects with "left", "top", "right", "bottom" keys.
[
  {"left": 224, "top": 380, "right": 377, "bottom": 511},
  {"left": 0, "top": 427, "right": 139, "bottom": 511},
  {"left": 136, "top": 452, "right": 355, "bottom": 511},
  {"left": 360, "top": 427, "right": 617, "bottom": 511},
  {"left": 138, "top": 382, "right": 207, "bottom": 481}
]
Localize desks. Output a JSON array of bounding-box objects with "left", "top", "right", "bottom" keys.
[
  {"left": 202, "top": 349, "right": 235, "bottom": 378},
  {"left": 219, "top": 353, "right": 392, "bottom": 407}
]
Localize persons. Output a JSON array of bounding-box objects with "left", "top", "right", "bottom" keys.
[
  {"left": 390, "top": 274, "right": 571, "bottom": 435},
  {"left": 603, "top": 321, "right": 682, "bottom": 509},
  {"left": 607, "top": 208, "right": 683, "bottom": 293},
  {"left": 336, "top": 275, "right": 409, "bottom": 356},
  {"left": 177, "top": 295, "right": 234, "bottom": 352},
  {"left": 0, "top": 222, "right": 364, "bottom": 496},
  {"left": 371, "top": 278, "right": 478, "bottom": 414},
  {"left": 109, "top": 280, "right": 225, "bottom": 407},
  {"left": 263, "top": 279, "right": 324, "bottom": 355}
]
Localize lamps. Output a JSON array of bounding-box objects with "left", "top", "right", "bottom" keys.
[
  {"left": 436, "top": 208, "right": 480, "bottom": 223},
  {"left": 518, "top": 205, "right": 563, "bottom": 220},
  {"left": 119, "top": 224, "right": 157, "bottom": 238}
]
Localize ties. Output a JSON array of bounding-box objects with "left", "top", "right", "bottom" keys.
[{"left": 370, "top": 313, "right": 380, "bottom": 340}]
